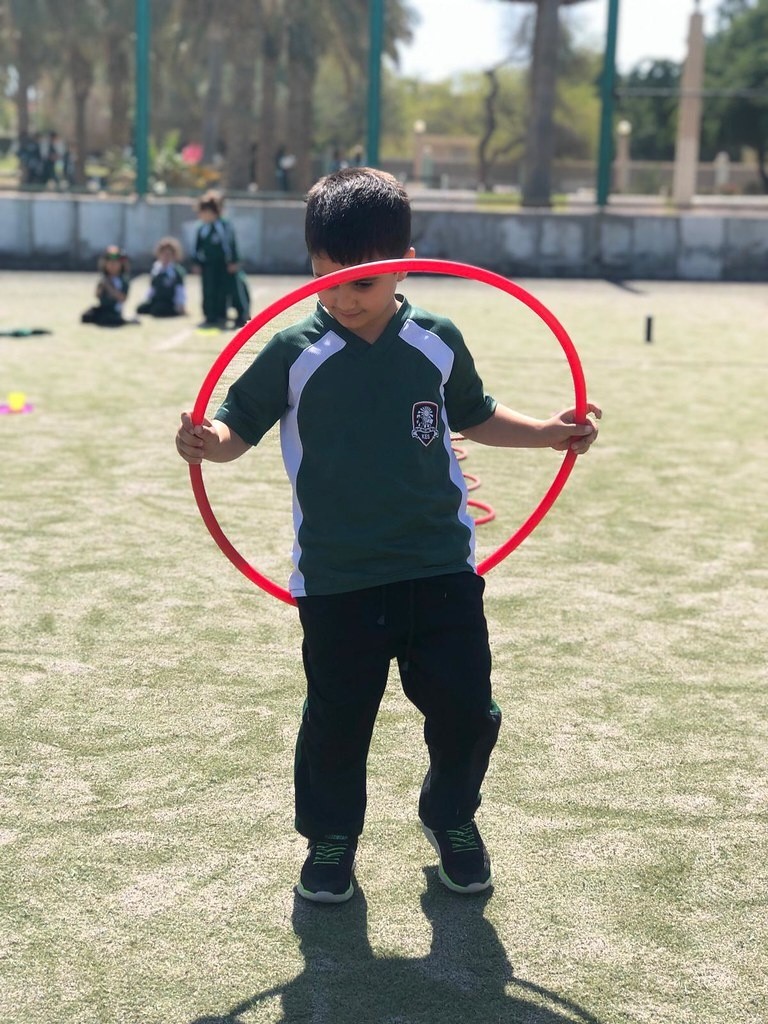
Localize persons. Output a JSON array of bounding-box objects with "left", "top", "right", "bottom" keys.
[
  {"left": 250, "top": 144, "right": 364, "bottom": 191},
  {"left": 16, "top": 131, "right": 74, "bottom": 192},
  {"left": 137, "top": 239, "right": 187, "bottom": 318},
  {"left": 83, "top": 246, "right": 131, "bottom": 327},
  {"left": 175, "top": 168, "right": 604, "bottom": 904},
  {"left": 194, "top": 191, "right": 251, "bottom": 329}
]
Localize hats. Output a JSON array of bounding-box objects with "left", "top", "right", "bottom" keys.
[
  {"left": 98, "top": 246, "right": 129, "bottom": 277},
  {"left": 190, "top": 189, "right": 225, "bottom": 216},
  {"left": 153, "top": 236, "right": 182, "bottom": 261}
]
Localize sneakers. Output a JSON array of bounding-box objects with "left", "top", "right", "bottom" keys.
[
  {"left": 296, "top": 837, "right": 358, "bottom": 903},
  {"left": 419, "top": 810, "right": 495, "bottom": 894}
]
